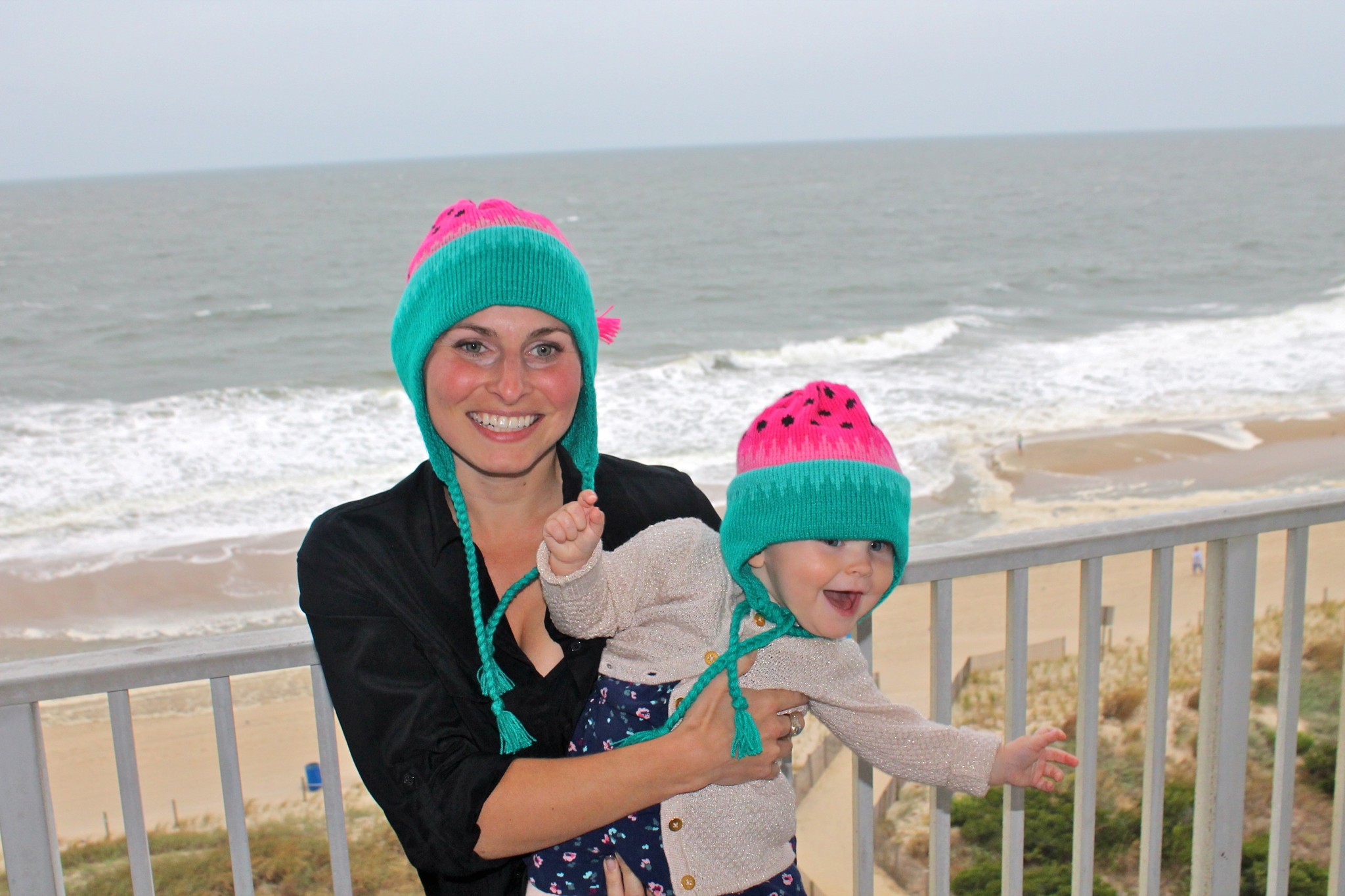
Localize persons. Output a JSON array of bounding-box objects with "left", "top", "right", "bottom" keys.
[
  {"left": 296, "top": 198, "right": 807, "bottom": 896},
  {"left": 522, "top": 380, "right": 1079, "bottom": 896}
]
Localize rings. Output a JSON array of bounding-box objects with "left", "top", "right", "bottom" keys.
[{"left": 783, "top": 713, "right": 802, "bottom": 737}]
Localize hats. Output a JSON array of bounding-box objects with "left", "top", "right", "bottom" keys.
[
  {"left": 388, "top": 198, "right": 622, "bottom": 754},
  {"left": 613, "top": 381, "right": 913, "bottom": 762}
]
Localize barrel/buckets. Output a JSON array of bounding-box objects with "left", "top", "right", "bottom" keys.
[{"left": 306, "top": 763, "right": 321, "bottom": 790}]
